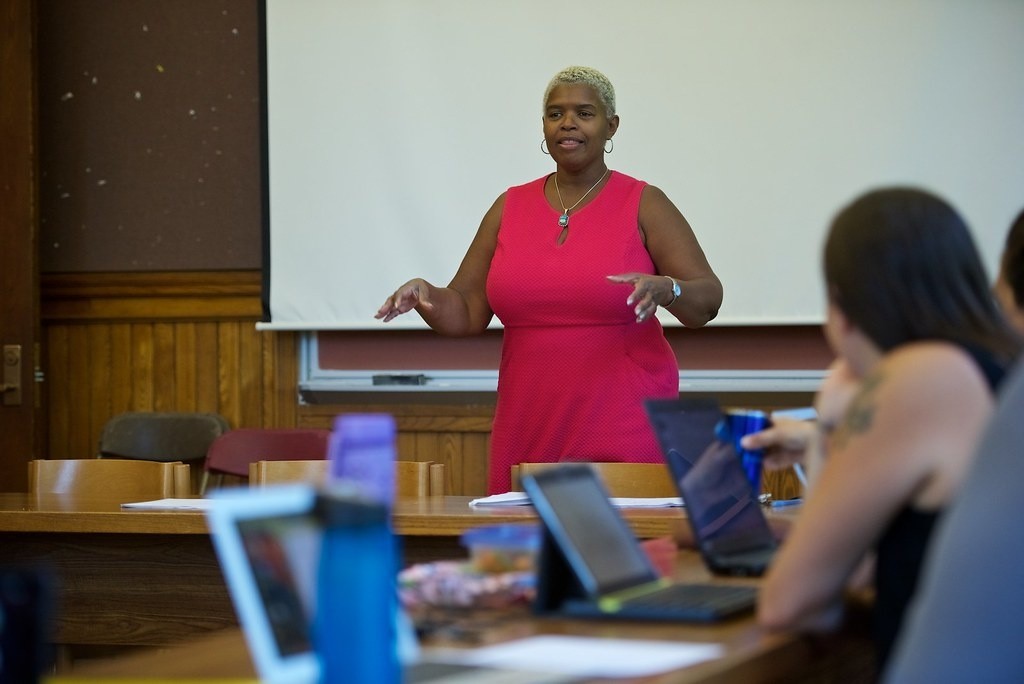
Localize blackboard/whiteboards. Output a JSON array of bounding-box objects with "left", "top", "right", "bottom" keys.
[{"left": 293, "top": 325, "right": 838, "bottom": 396}]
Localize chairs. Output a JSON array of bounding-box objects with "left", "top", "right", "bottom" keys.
[
  {"left": 248, "top": 459, "right": 447, "bottom": 497},
  {"left": 510, "top": 462, "right": 680, "bottom": 499},
  {"left": 97, "top": 413, "right": 232, "bottom": 490},
  {"left": 27, "top": 459, "right": 193, "bottom": 499},
  {"left": 198, "top": 428, "right": 331, "bottom": 496}
]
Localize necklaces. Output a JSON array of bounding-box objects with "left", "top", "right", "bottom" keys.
[{"left": 554, "top": 169, "right": 608, "bottom": 226}]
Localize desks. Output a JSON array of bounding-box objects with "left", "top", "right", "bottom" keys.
[
  {"left": 56, "top": 547, "right": 796, "bottom": 684},
  {"left": 0, "top": 493, "right": 802, "bottom": 675}
]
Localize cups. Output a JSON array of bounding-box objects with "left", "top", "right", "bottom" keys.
[{"left": 329, "top": 414, "right": 395, "bottom": 484}]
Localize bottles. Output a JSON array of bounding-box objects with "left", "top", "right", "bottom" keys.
[
  {"left": 729, "top": 409, "right": 769, "bottom": 494},
  {"left": 307, "top": 483, "right": 404, "bottom": 684}
]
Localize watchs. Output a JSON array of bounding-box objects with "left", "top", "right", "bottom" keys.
[{"left": 661, "top": 276, "right": 681, "bottom": 309}]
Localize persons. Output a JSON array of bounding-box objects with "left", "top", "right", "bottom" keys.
[
  {"left": 374, "top": 67, "right": 723, "bottom": 495},
  {"left": 740, "top": 188, "right": 1024, "bottom": 684}
]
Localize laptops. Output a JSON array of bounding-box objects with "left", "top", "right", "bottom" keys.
[
  {"left": 520, "top": 462, "right": 761, "bottom": 622},
  {"left": 644, "top": 397, "right": 780, "bottom": 577},
  {"left": 771, "top": 407, "right": 818, "bottom": 486},
  {"left": 207, "top": 481, "right": 581, "bottom": 684}
]
